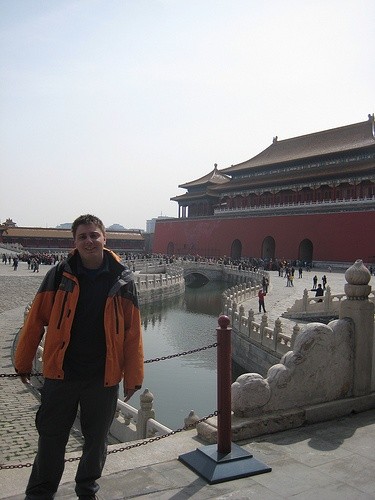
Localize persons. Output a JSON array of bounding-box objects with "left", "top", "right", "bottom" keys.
[
  {"left": 328, "top": 266, "right": 332, "bottom": 272},
  {"left": 345, "top": 263, "right": 375, "bottom": 277},
  {"left": 0, "top": 248, "right": 310, "bottom": 291},
  {"left": 14, "top": 214, "right": 145, "bottom": 500},
  {"left": 309, "top": 283, "right": 324, "bottom": 303},
  {"left": 322, "top": 275, "right": 327, "bottom": 289},
  {"left": 258, "top": 290, "right": 267, "bottom": 313},
  {"left": 313, "top": 275, "right": 318, "bottom": 290}
]
